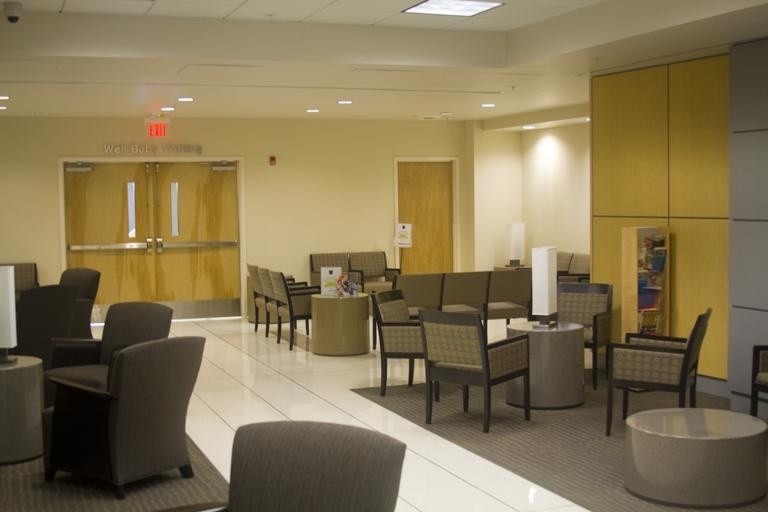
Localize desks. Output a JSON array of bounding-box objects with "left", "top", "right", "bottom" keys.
[
  {"left": 502, "top": 319, "right": 589, "bottom": 413},
  {"left": 0, "top": 356, "right": 44, "bottom": 464},
  {"left": 622, "top": 407, "right": 768, "bottom": 509}
]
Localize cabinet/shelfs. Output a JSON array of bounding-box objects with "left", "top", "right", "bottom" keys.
[{"left": 620, "top": 225, "right": 672, "bottom": 341}]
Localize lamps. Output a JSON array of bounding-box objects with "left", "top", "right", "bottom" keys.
[
  {"left": 506, "top": 222, "right": 526, "bottom": 268},
  {"left": 527, "top": 245, "right": 561, "bottom": 328}
]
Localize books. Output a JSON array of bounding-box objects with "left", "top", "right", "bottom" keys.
[{"left": 638, "top": 234, "right": 667, "bottom": 334}]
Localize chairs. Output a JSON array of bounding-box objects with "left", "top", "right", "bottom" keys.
[
  {"left": 540, "top": 280, "right": 611, "bottom": 390},
  {"left": 415, "top": 307, "right": 534, "bottom": 432},
  {"left": 247, "top": 247, "right": 533, "bottom": 353},
  {"left": 40, "top": 336, "right": 205, "bottom": 499},
  {"left": 540, "top": 249, "right": 593, "bottom": 281},
  {"left": 159, "top": 421, "right": 407, "bottom": 512},
  {"left": 44, "top": 301, "right": 173, "bottom": 403},
  {"left": 606, "top": 307, "right": 712, "bottom": 435},
  {"left": 0, "top": 263, "right": 36, "bottom": 305},
  {"left": 16, "top": 284, "right": 77, "bottom": 373},
  {"left": 370, "top": 289, "right": 443, "bottom": 404},
  {"left": 57, "top": 267, "right": 100, "bottom": 339},
  {"left": 751, "top": 345, "right": 768, "bottom": 418}
]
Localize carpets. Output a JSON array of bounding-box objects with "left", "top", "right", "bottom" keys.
[
  {"left": 0, "top": 428, "right": 230, "bottom": 512},
  {"left": 349, "top": 366, "right": 768, "bottom": 511}
]
